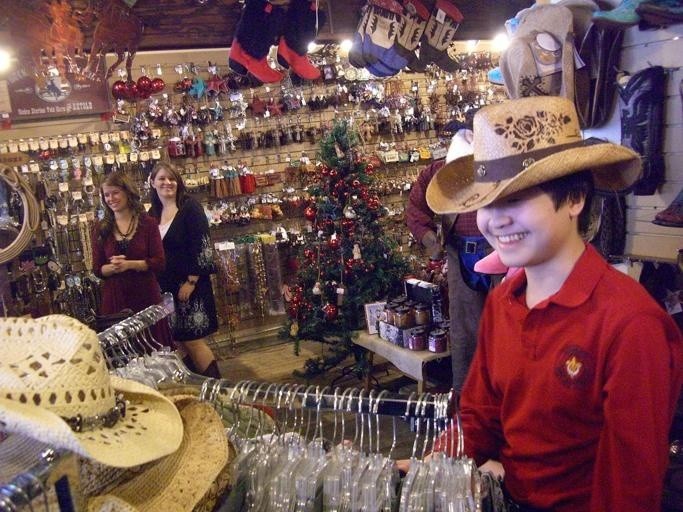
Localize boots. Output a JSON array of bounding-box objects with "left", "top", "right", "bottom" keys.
[
  {"left": 652, "top": 189, "right": 683, "bottom": 227},
  {"left": 228, "top": 0, "right": 284, "bottom": 82},
  {"left": 597, "top": 66, "right": 664, "bottom": 195},
  {"left": 278, "top": 0, "right": 321, "bottom": 79}
]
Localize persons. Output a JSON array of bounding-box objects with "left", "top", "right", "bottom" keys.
[
  {"left": 146, "top": 159, "right": 224, "bottom": 379},
  {"left": 403, "top": 106, "right": 504, "bottom": 398},
  {"left": 389, "top": 95, "right": 683, "bottom": 512},
  {"left": 89, "top": 170, "right": 177, "bottom": 354}
]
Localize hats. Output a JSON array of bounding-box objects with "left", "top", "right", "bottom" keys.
[
  {"left": 0, "top": 394, "right": 228, "bottom": 512},
  {"left": 499, "top": 3, "right": 577, "bottom": 101},
  {"left": 0, "top": 312, "right": 184, "bottom": 468},
  {"left": 425, "top": 95, "right": 642, "bottom": 215}
]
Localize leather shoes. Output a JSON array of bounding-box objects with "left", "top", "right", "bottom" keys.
[
  {"left": 594, "top": 0, "right": 657, "bottom": 32},
  {"left": 635, "top": 0, "right": 683, "bottom": 21}
]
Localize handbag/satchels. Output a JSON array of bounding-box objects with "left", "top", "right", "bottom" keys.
[{"left": 449, "top": 233, "right": 490, "bottom": 292}]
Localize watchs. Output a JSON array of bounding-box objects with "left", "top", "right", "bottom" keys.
[{"left": 186, "top": 277, "right": 197, "bottom": 286}]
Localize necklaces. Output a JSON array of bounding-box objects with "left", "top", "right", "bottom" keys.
[{"left": 114, "top": 213, "right": 136, "bottom": 247}]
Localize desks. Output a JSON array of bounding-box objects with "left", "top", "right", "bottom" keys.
[{"left": 350, "top": 329, "right": 450, "bottom": 395}]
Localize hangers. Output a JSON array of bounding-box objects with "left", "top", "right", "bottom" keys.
[
  {"left": 0, "top": 471, "right": 51, "bottom": 512},
  {"left": 95, "top": 303, "right": 211, "bottom": 390},
  {"left": 200, "top": 378, "right": 484, "bottom": 512}
]
